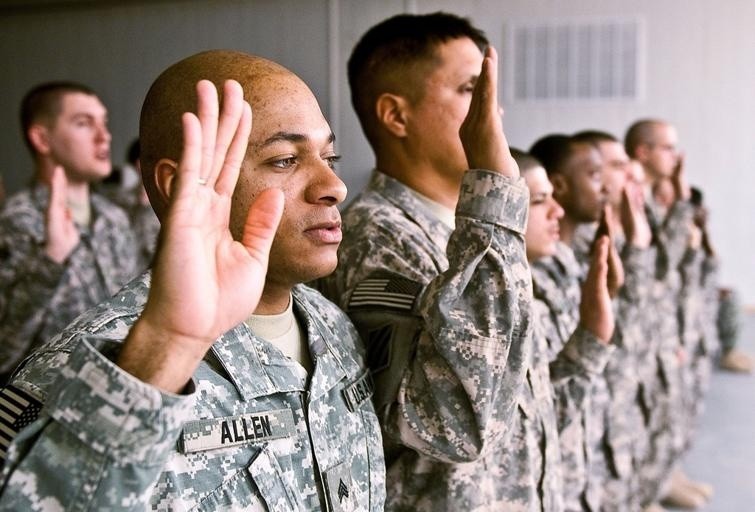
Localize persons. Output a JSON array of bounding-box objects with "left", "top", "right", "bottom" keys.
[
  {"left": 626, "top": 115, "right": 750, "bottom": 512},
  {"left": 0, "top": 82, "right": 139, "bottom": 378},
  {"left": 578, "top": 130, "right": 657, "bottom": 512},
  {"left": 507, "top": 143, "right": 617, "bottom": 512},
  {"left": 121, "top": 138, "right": 161, "bottom": 271},
  {"left": 0, "top": 46, "right": 388, "bottom": 512},
  {"left": 310, "top": 8, "right": 566, "bottom": 512},
  {"left": 530, "top": 129, "right": 626, "bottom": 512}
]
